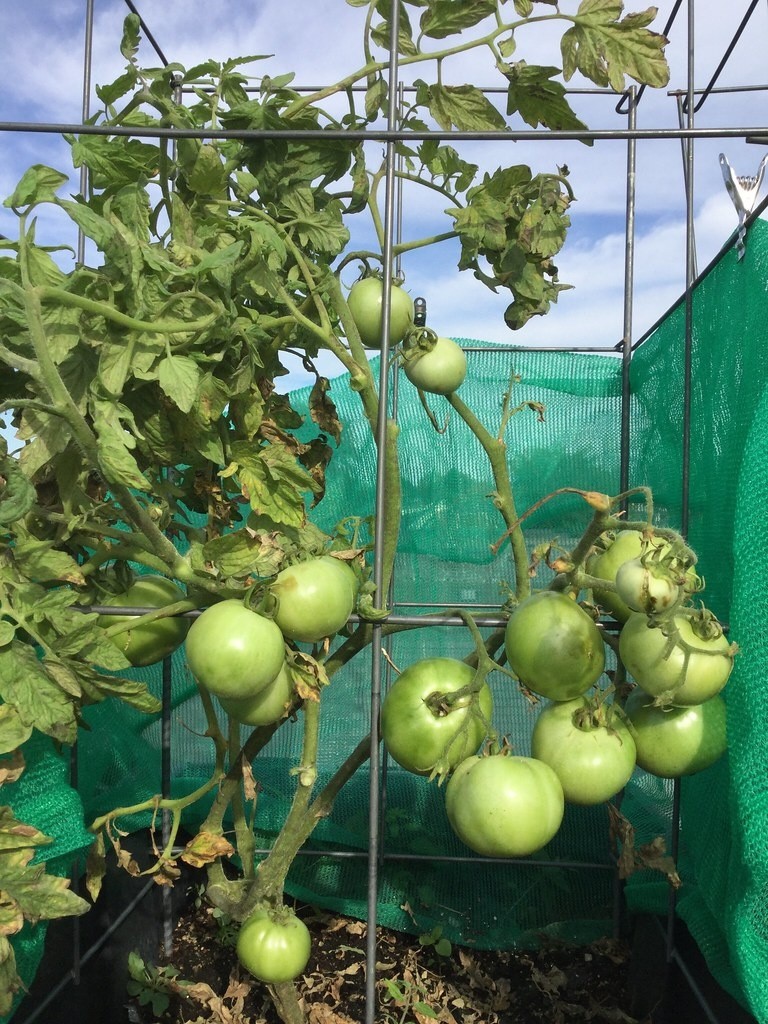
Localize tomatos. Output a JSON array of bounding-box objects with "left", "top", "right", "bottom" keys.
[
  {"left": 378, "top": 530, "right": 733, "bottom": 858},
  {"left": 237, "top": 908, "right": 312, "bottom": 983},
  {"left": 86, "top": 274, "right": 466, "bottom": 727}
]
